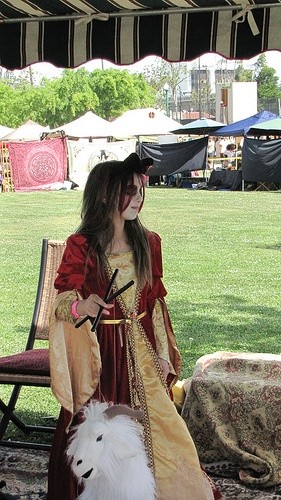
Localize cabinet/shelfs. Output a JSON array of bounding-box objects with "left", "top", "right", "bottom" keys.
[{"left": 0, "top": 138, "right": 23, "bottom": 192}]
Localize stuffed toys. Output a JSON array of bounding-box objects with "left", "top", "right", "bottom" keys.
[{"left": 64, "top": 399, "right": 156, "bottom": 500}]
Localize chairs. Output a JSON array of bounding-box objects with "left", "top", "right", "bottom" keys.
[{"left": 0, "top": 239, "right": 68, "bottom": 450}]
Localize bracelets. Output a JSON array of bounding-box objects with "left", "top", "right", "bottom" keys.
[{"left": 72, "top": 300, "right": 80, "bottom": 319}]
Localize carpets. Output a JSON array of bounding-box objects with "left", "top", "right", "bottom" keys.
[{"left": 0, "top": 445, "right": 281, "bottom": 500}]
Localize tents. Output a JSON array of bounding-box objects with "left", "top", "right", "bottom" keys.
[
  {"left": 0, "top": 106, "right": 181, "bottom": 187},
  {"left": 169, "top": 118, "right": 228, "bottom": 137},
  {"left": 209, "top": 109, "right": 279, "bottom": 169},
  {"left": 242, "top": 118, "right": 281, "bottom": 192}
]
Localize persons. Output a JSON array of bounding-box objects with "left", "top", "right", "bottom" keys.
[
  {"left": 45, "top": 160, "right": 221, "bottom": 500},
  {"left": 206, "top": 136, "right": 241, "bottom": 170}
]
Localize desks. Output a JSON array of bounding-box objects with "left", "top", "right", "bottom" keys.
[
  {"left": 179, "top": 177, "right": 208, "bottom": 188},
  {"left": 207, "top": 170, "right": 257, "bottom": 192}
]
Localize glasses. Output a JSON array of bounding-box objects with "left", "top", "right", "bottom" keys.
[{"left": 121, "top": 185, "right": 144, "bottom": 197}]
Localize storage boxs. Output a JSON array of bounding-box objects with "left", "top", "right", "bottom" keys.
[{"left": 192, "top": 183, "right": 198, "bottom": 189}]
